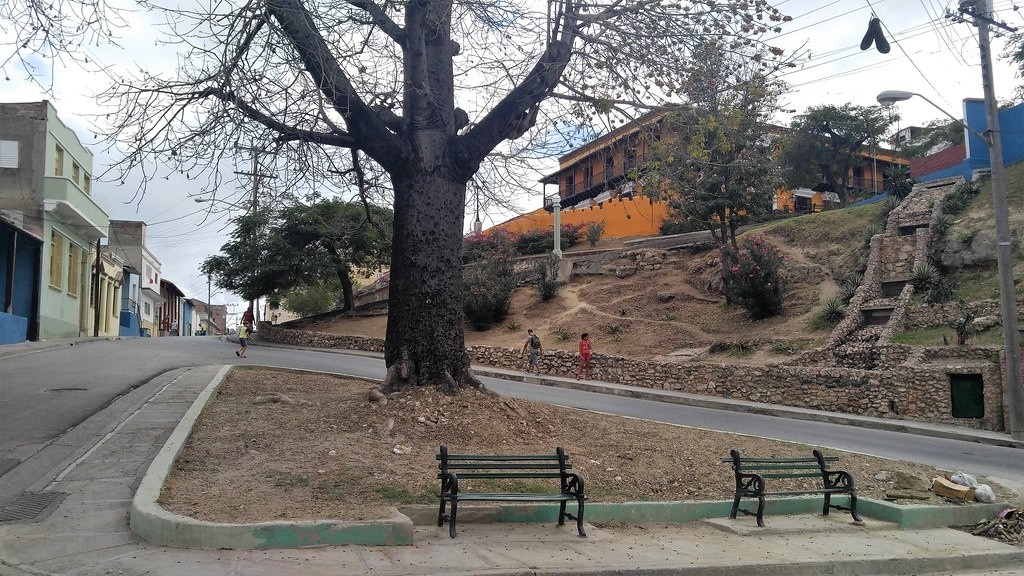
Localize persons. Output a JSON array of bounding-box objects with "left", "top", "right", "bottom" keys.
[
  {"left": 577, "top": 332, "right": 592, "bottom": 380},
  {"left": 199, "top": 323, "right": 206, "bottom": 336},
  {"left": 240, "top": 307, "right": 255, "bottom": 335},
  {"left": 235, "top": 321, "right": 253, "bottom": 358},
  {"left": 521, "top": 329, "right": 544, "bottom": 376},
  {"left": 170, "top": 320, "right": 178, "bottom": 336}
]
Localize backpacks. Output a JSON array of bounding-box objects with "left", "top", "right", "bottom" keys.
[{"left": 529, "top": 334, "right": 541, "bottom": 349}]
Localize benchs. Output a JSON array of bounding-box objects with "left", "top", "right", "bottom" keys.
[
  {"left": 436, "top": 445, "right": 587, "bottom": 538},
  {"left": 720, "top": 449, "right": 864, "bottom": 527}
]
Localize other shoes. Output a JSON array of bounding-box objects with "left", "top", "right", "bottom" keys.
[
  {"left": 525, "top": 369, "right": 529, "bottom": 374},
  {"left": 241, "top": 355, "right": 247, "bottom": 358},
  {"left": 536, "top": 372, "right": 542, "bottom": 377},
  {"left": 235, "top": 351, "right": 240, "bottom": 356}
]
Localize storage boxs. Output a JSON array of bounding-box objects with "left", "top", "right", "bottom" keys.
[{"left": 934, "top": 474, "right": 975, "bottom": 501}]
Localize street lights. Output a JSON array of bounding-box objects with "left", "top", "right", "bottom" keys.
[
  {"left": 193, "top": 197, "right": 258, "bottom": 310},
  {"left": 874, "top": 89, "right": 1024, "bottom": 440},
  {"left": 207, "top": 291, "right": 223, "bottom": 336}
]
